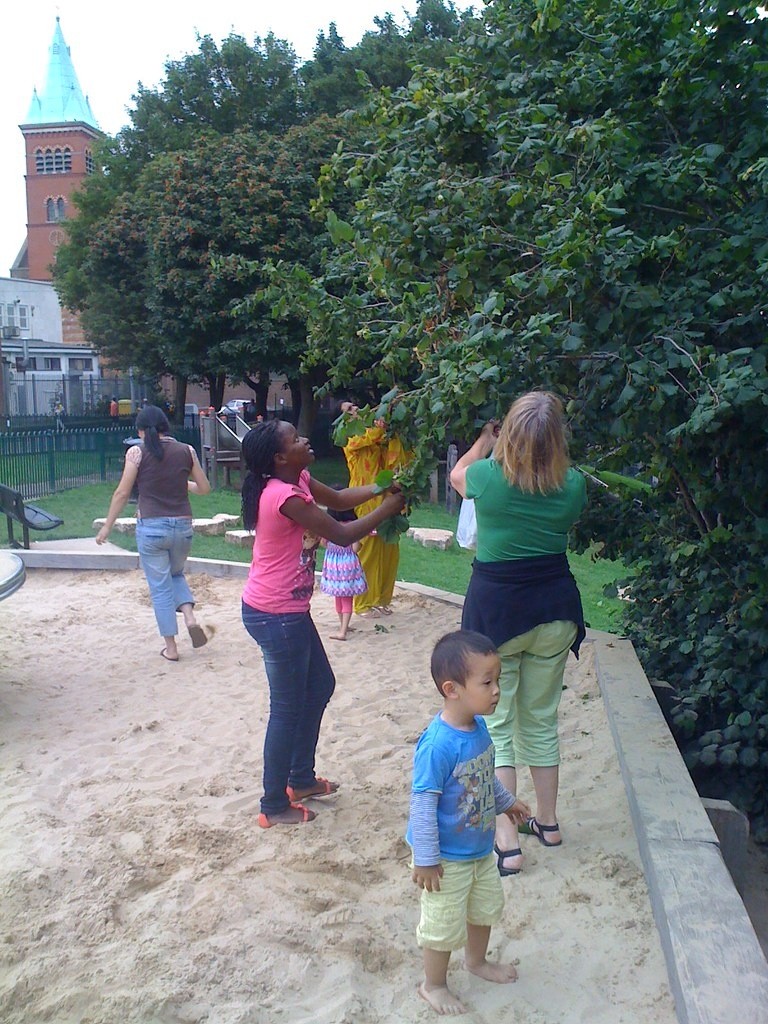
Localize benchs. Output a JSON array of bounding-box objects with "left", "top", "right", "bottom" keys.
[{"left": 0, "top": 483, "right": 64, "bottom": 549}]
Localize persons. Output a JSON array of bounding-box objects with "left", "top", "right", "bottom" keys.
[
  {"left": 239, "top": 418, "right": 408, "bottom": 829},
  {"left": 54, "top": 400, "right": 67, "bottom": 433},
  {"left": 247, "top": 399, "right": 258, "bottom": 420},
  {"left": 331, "top": 384, "right": 416, "bottom": 616},
  {"left": 96, "top": 406, "right": 212, "bottom": 660},
  {"left": 319, "top": 483, "right": 369, "bottom": 642},
  {"left": 110, "top": 397, "right": 119, "bottom": 416},
  {"left": 404, "top": 630, "right": 531, "bottom": 1014},
  {"left": 451, "top": 389, "right": 588, "bottom": 875}
]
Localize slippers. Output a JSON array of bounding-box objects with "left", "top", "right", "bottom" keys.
[
  {"left": 359, "top": 608, "right": 380, "bottom": 618},
  {"left": 376, "top": 605, "right": 393, "bottom": 615},
  {"left": 161, "top": 647, "right": 179, "bottom": 661},
  {"left": 188, "top": 625, "right": 207, "bottom": 648}
]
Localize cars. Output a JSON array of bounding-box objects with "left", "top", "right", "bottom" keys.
[{"left": 218, "top": 399, "right": 252, "bottom": 415}]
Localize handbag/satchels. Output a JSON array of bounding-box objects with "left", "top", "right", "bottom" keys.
[{"left": 455, "top": 448, "right": 495, "bottom": 551}]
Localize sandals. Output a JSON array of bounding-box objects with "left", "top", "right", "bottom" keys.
[
  {"left": 259, "top": 801, "right": 317, "bottom": 829},
  {"left": 286, "top": 777, "right": 338, "bottom": 801},
  {"left": 518, "top": 817, "right": 562, "bottom": 846},
  {"left": 493, "top": 844, "right": 523, "bottom": 876}
]
